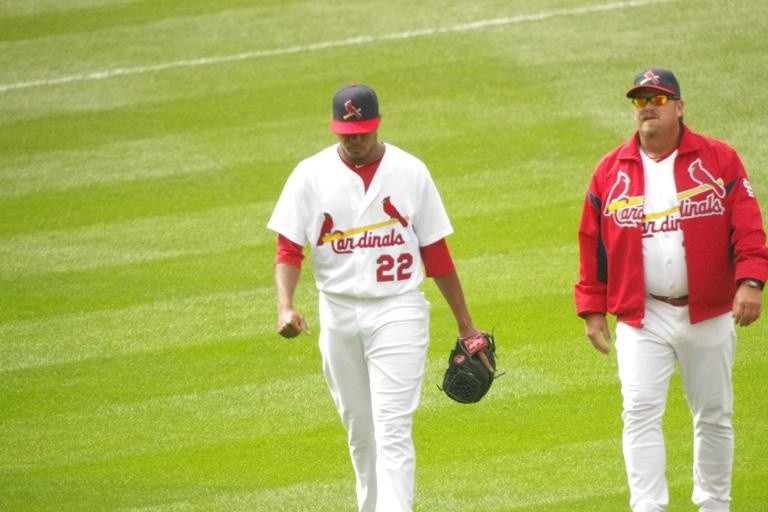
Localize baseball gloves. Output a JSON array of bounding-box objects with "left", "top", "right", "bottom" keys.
[{"left": 443, "top": 332, "right": 496, "bottom": 403}]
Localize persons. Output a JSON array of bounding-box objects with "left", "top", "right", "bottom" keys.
[
  {"left": 266, "top": 85, "right": 495, "bottom": 512},
  {"left": 575, "top": 69, "right": 768, "bottom": 512}
]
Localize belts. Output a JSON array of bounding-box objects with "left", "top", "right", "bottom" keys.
[{"left": 649, "top": 292, "right": 690, "bottom": 308}]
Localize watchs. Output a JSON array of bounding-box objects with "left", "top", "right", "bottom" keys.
[{"left": 738, "top": 279, "right": 763, "bottom": 292}]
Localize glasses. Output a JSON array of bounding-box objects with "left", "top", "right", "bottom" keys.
[{"left": 631, "top": 93, "right": 674, "bottom": 107}]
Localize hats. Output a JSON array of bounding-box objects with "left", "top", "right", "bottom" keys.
[
  {"left": 625, "top": 67, "right": 682, "bottom": 99},
  {"left": 327, "top": 83, "right": 382, "bottom": 135}
]
